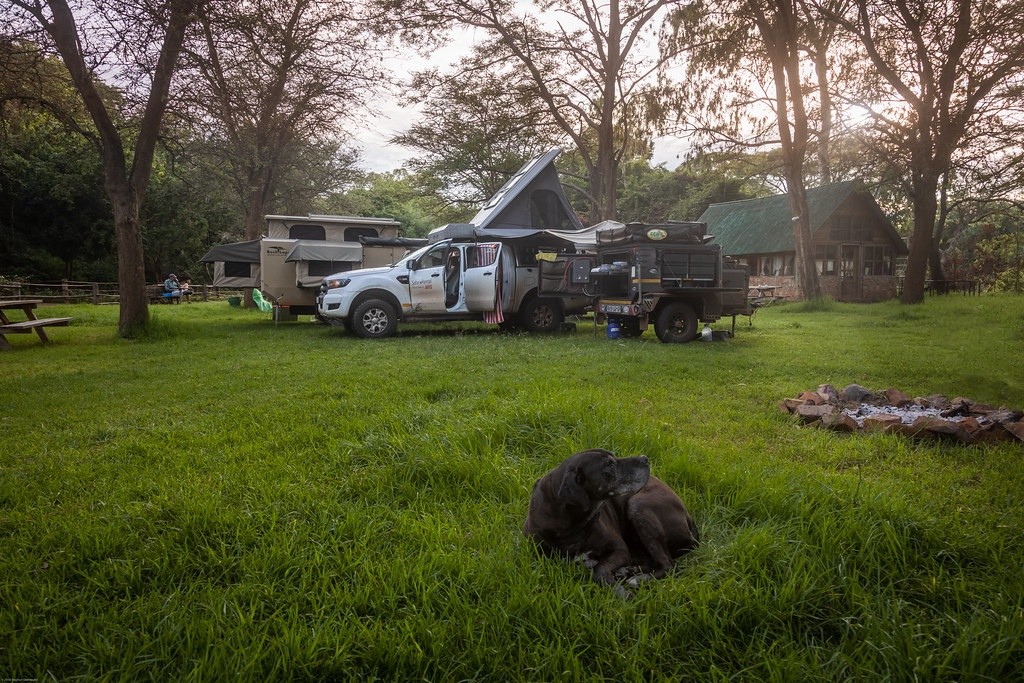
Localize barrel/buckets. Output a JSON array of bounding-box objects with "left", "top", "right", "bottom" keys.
[
  {"left": 228, "top": 296, "right": 242, "bottom": 307},
  {"left": 607, "top": 320, "right": 619, "bottom": 339}
]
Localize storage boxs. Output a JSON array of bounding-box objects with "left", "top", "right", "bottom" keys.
[{"left": 273, "top": 306, "right": 297, "bottom": 321}]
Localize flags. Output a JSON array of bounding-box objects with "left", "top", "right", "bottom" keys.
[{"left": 478, "top": 244, "right": 507, "bottom": 324}]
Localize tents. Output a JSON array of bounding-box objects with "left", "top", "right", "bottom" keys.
[
  {"left": 472, "top": 146, "right": 587, "bottom": 230},
  {"left": 254, "top": 212, "right": 429, "bottom": 312},
  {"left": 198, "top": 236, "right": 261, "bottom": 287}
]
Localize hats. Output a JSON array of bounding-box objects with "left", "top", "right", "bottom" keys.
[{"left": 169, "top": 274, "right": 177, "bottom": 278}]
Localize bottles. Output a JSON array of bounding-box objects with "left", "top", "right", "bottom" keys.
[{"left": 701, "top": 324, "right": 712, "bottom": 341}]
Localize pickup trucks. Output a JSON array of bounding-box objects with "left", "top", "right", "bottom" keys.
[{"left": 319, "top": 222, "right": 630, "bottom": 339}]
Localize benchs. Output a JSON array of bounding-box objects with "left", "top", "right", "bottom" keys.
[
  {"left": 0, "top": 316, "right": 75, "bottom": 334},
  {"left": 148, "top": 286, "right": 180, "bottom": 304},
  {"left": 747, "top": 295, "right": 790, "bottom": 301}
]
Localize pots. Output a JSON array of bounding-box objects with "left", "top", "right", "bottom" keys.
[{"left": 712, "top": 330, "right": 728, "bottom": 339}]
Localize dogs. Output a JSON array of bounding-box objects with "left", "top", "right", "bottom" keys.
[{"left": 522, "top": 449, "right": 699, "bottom": 600}]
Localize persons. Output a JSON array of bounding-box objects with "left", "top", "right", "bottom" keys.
[{"left": 164, "top": 274, "right": 193, "bottom": 304}]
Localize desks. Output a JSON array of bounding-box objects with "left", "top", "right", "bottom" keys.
[
  {"left": 0, "top": 300, "right": 52, "bottom": 350},
  {"left": 749, "top": 285, "right": 782, "bottom": 306}
]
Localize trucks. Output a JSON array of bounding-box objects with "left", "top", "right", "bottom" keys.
[{"left": 586, "top": 218, "right": 759, "bottom": 343}]
